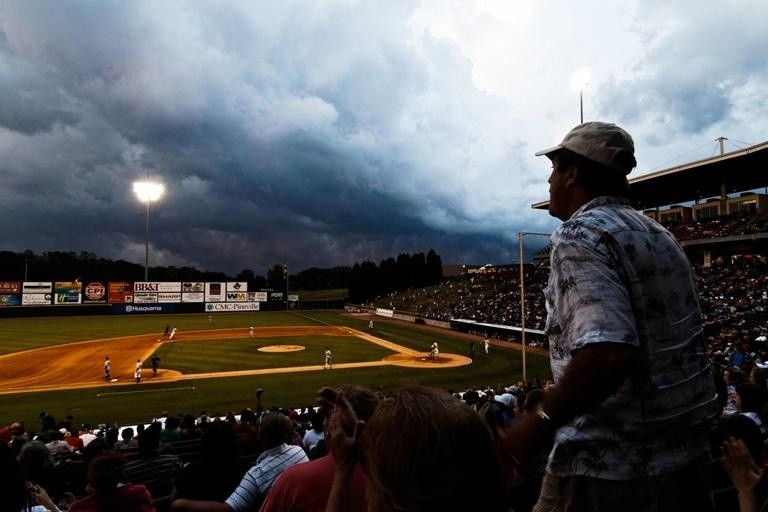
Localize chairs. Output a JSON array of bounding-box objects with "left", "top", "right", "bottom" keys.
[{"left": 1, "top": 378, "right": 562, "bottom": 511}]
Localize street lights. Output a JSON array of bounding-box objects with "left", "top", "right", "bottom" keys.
[{"left": 128, "top": 176, "right": 166, "bottom": 281}]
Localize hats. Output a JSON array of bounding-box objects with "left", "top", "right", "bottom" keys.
[
  {"left": 503, "top": 385, "right": 519, "bottom": 394},
  {"left": 494, "top": 393, "right": 516, "bottom": 409},
  {"left": 535, "top": 123, "right": 636, "bottom": 175}
]
